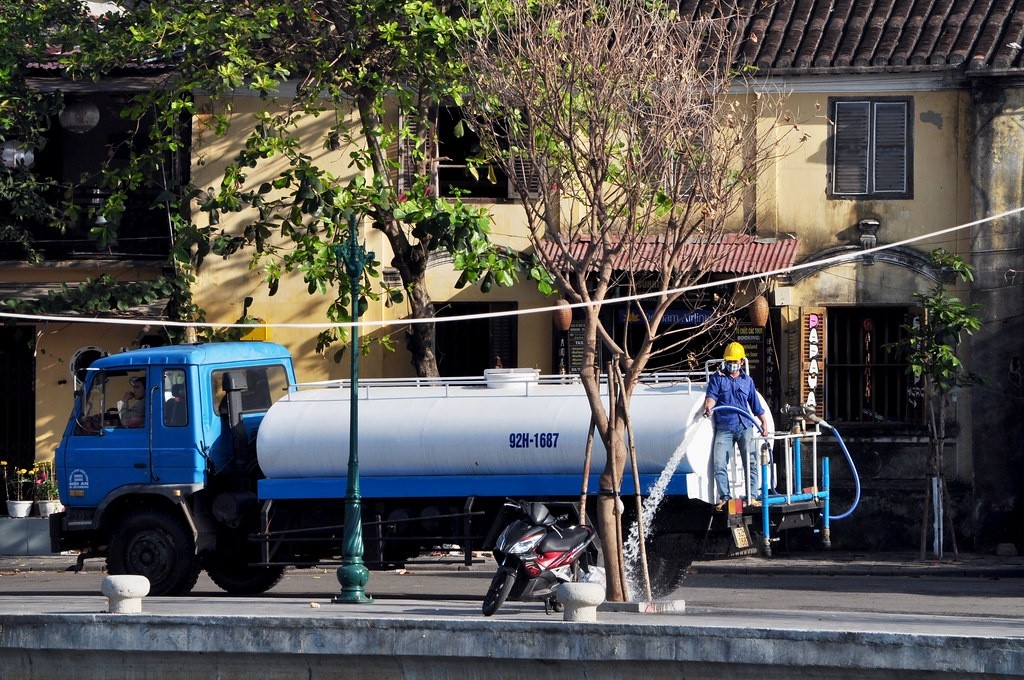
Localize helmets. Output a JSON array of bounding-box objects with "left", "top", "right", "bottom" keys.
[{"left": 723, "top": 341, "right": 746, "bottom": 361}]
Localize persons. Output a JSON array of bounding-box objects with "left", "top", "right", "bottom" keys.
[
  {"left": 703, "top": 341, "right": 768, "bottom": 511},
  {"left": 116, "top": 376, "right": 146, "bottom": 427}
]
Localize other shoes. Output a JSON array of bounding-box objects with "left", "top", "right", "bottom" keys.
[
  {"left": 745, "top": 498, "right": 760, "bottom": 507},
  {"left": 716, "top": 503, "right": 728, "bottom": 511}
]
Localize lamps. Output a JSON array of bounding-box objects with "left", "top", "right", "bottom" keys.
[
  {"left": 553, "top": 295, "right": 573, "bottom": 330},
  {"left": 749, "top": 295, "right": 769, "bottom": 327}
]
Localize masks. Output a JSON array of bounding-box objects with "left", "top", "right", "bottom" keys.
[{"left": 725, "top": 363, "right": 741, "bottom": 374}]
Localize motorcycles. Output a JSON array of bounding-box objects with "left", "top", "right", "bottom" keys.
[{"left": 482, "top": 497, "right": 597, "bottom": 616}]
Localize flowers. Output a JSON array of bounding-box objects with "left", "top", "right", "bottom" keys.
[
  {"left": 1, "top": 461, "right": 35, "bottom": 501},
  {"left": 33, "top": 461, "right": 60, "bottom": 501}
]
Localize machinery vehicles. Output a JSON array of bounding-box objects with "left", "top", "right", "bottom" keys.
[{"left": 49, "top": 341, "right": 861, "bottom": 598}]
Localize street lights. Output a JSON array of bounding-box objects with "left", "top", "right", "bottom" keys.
[{"left": 329, "top": 197, "right": 372, "bottom": 604}]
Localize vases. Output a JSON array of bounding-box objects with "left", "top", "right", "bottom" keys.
[
  {"left": 6, "top": 501, "right": 34, "bottom": 518},
  {"left": 36, "top": 500, "right": 63, "bottom": 518}
]
